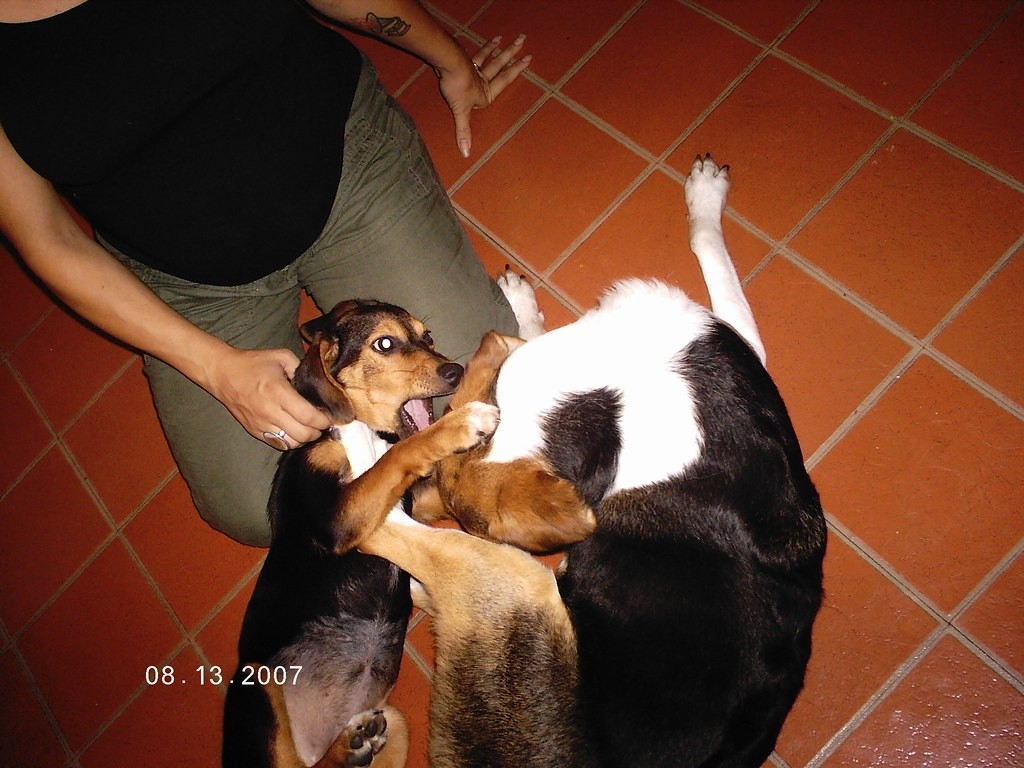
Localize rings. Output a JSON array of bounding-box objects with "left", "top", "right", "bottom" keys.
[
  {"left": 473, "top": 63, "right": 478, "bottom": 66},
  {"left": 263, "top": 430, "right": 291, "bottom": 451}
]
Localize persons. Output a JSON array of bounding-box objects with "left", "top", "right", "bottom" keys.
[{"left": 0, "top": 0, "right": 533, "bottom": 548}]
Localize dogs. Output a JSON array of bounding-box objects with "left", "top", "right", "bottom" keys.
[{"left": 221, "top": 150, "right": 828, "bottom": 768}]
